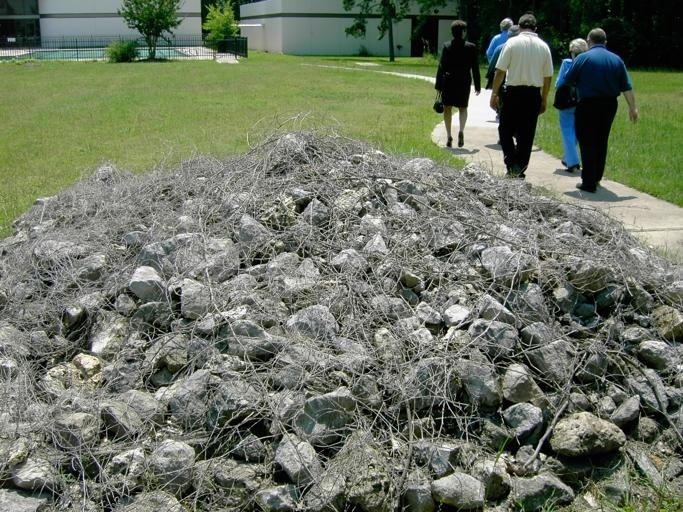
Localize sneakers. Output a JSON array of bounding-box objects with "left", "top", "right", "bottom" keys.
[
  {"left": 560, "top": 158, "right": 582, "bottom": 171},
  {"left": 575, "top": 181, "right": 597, "bottom": 193}
]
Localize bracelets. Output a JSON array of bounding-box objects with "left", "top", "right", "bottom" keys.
[{"left": 491, "top": 94, "right": 497, "bottom": 96}]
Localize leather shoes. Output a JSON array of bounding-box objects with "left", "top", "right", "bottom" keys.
[
  {"left": 446, "top": 135, "right": 453, "bottom": 148},
  {"left": 458, "top": 131, "right": 464, "bottom": 146}
]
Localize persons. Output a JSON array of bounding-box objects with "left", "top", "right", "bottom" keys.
[
  {"left": 490, "top": 13, "right": 553, "bottom": 179},
  {"left": 553, "top": 38, "right": 590, "bottom": 175},
  {"left": 435, "top": 20, "right": 481, "bottom": 148},
  {"left": 562, "top": 27, "right": 638, "bottom": 195},
  {"left": 485, "top": 17, "right": 513, "bottom": 63},
  {"left": 484, "top": 26, "right": 520, "bottom": 145}
]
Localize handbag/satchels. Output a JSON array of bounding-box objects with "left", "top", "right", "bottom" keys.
[
  {"left": 432, "top": 87, "right": 445, "bottom": 113},
  {"left": 551, "top": 79, "right": 578, "bottom": 110}
]
